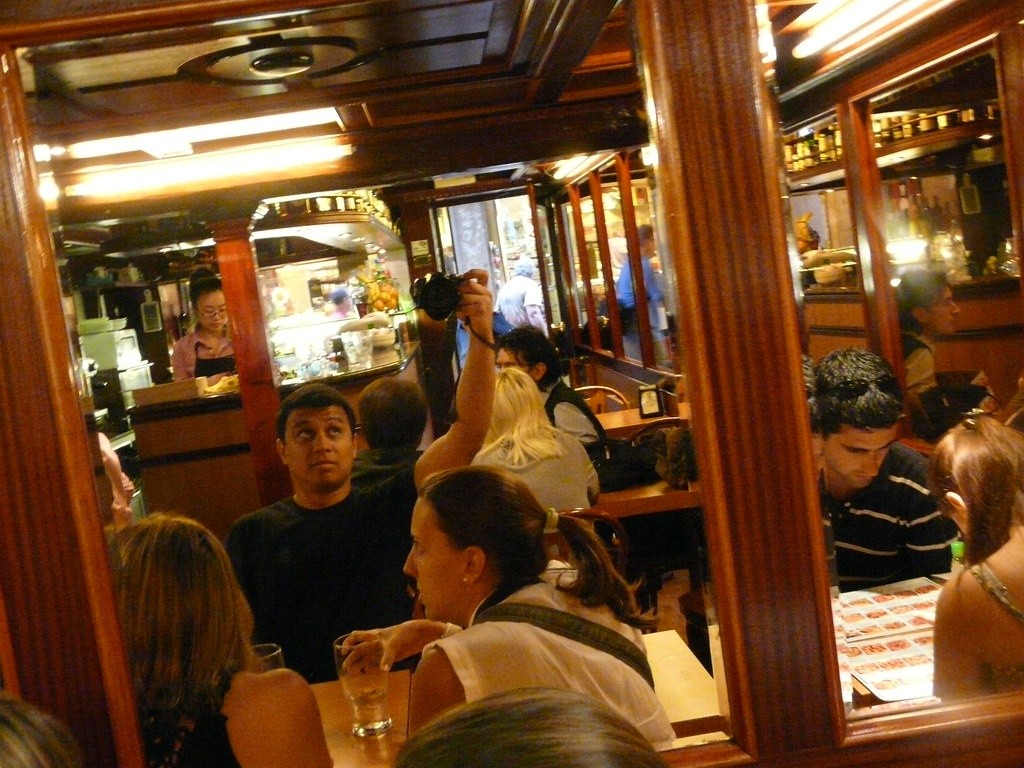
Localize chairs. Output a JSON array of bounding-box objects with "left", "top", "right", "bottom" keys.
[
  {"left": 541, "top": 504, "right": 628, "bottom": 595},
  {"left": 574, "top": 384, "right": 628, "bottom": 417},
  {"left": 623, "top": 416, "right": 686, "bottom": 464}
]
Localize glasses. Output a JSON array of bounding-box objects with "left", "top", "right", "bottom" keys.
[
  {"left": 195, "top": 305, "right": 228, "bottom": 319},
  {"left": 353, "top": 422, "right": 364, "bottom": 433},
  {"left": 496, "top": 362, "right": 528, "bottom": 371},
  {"left": 816, "top": 374, "right": 902, "bottom": 402}
]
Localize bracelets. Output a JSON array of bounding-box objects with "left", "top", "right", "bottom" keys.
[{"left": 443, "top": 622, "right": 463, "bottom": 639}]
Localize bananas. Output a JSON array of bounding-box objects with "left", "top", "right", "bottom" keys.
[{"left": 366, "top": 282, "right": 379, "bottom": 304}]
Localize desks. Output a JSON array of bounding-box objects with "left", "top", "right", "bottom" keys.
[{"left": 297, "top": 401, "right": 1024, "bottom": 768}]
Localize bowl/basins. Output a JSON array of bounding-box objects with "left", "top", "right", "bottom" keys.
[
  {"left": 373, "top": 334, "right": 395, "bottom": 347},
  {"left": 814, "top": 272, "right": 846, "bottom": 282}
]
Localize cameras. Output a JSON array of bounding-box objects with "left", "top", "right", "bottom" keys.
[{"left": 412, "top": 269, "right": 467, "bottom": 320}]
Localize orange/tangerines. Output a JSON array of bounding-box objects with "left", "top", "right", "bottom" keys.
[{"left": 372, "top": 286, "right": 398, "bottom": 310}]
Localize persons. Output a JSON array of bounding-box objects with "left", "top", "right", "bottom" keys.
[
  {"left": 492, "top": 258, "right": 554, "bottom": 339},
  {"left": 0, "top": 162, "right": 1024, "bottom": 768},
  {"left": 343, "top": 464, "right": 676, "bottom": 753},
  {"left": 806, "top": 345, "right": 951, "bottom": 579},
  {"left": 329, "top": 285, "right": 355, "bottom": 318},
  {"left": 497, "top": 324, "right": 601, "bottom": 450},
  {"left": 194, "top": 270, "right": 499, "bottom": 688},
  {"left": 103, "top": 512, "right": 333, "bottom": 768},
  {"left": 929, "top": 411, "right": 1024, "bottom": 702},
  {"left": 888, "top": 257, "right": 970, "bottom": 421},
  {"left": 173, "top": 260, "right": 242, "bottom": 380},
  {"left": 619, "top": 225, "right": 671, "bottom": 368},
  {"left": 468, "top": 365, "right": 597, "bottom": 523}
]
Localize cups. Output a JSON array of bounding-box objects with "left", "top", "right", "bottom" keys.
[
  {"left": 341, "top": 330, "right": 373, "bottom": 367},
  {"left": 251, "top": 644, "right": 284, "bottom": 670},
  {"left": 119, "top": 262, "right": 138, "bottom": 282},
  {"left": 333, "top": 634, "right": 394, "bottom": 736}
]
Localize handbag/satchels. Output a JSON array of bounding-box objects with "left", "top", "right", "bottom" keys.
[{"left": 587, "top": 436, "right": 636, "bottom": 493}]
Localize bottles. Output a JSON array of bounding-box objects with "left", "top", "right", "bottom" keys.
[
  {"left": 886, "top": 195, "right": 952, "bottom": 238},
  {"left": 950, "top": 541, "right": 964, "bottom": 569},
  {"left": 141, "top": 289, "right": 162, "bottom": 332},
  {"left": 784, "top": 104, "right": 994, "bottom": 172},
  {"left": 960, "top": 174, "right": 981, "bottom": 214}
]
